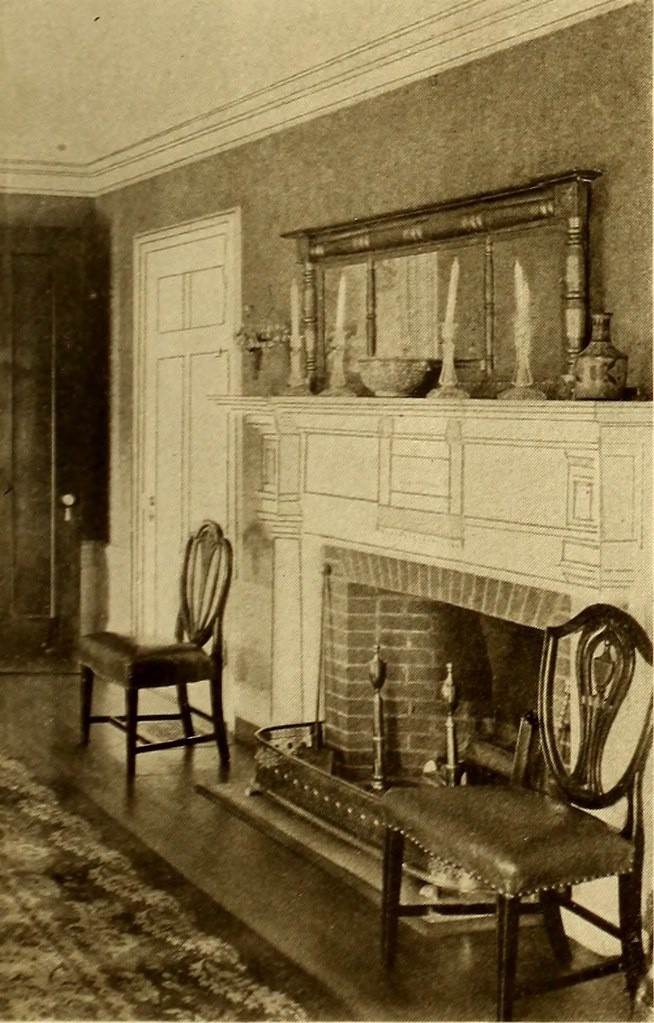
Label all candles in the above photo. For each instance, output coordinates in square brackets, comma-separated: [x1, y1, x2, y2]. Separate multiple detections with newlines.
[444, 254, 459, 337]
[335, 276, 346, 350]
[291, 278, 300, 346]
[514, 262, 535, 347]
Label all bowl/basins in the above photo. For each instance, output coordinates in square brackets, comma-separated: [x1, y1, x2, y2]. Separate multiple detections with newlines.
[358, 358, 433, 396]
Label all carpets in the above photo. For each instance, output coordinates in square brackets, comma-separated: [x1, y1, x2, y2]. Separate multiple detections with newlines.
[0, 722, 364, 1021]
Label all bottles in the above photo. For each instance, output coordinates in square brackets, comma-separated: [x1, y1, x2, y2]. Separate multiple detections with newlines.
[573, 314, 629, 399]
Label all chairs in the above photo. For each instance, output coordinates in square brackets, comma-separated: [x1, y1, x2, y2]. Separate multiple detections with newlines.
[382, 602, 654, 1022]
[79, 520, 234, 784]
